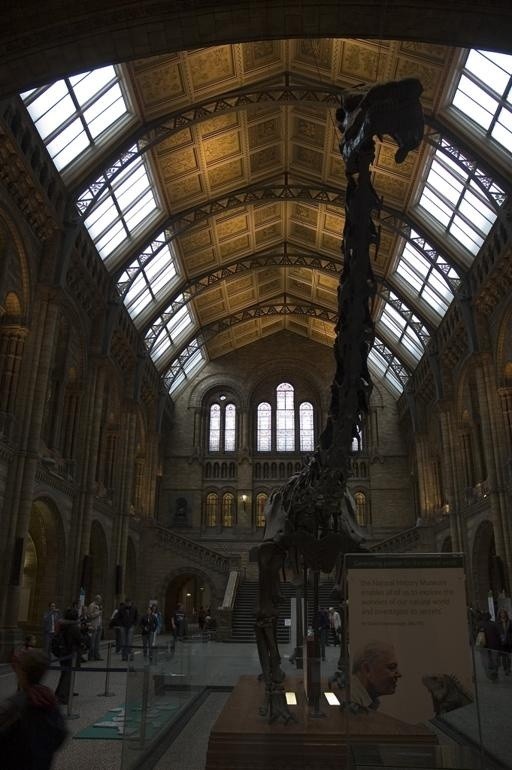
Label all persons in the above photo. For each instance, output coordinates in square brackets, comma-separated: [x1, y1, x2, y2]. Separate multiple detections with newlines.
[44, 593, 214, 667]
[348, 635, 404, 712]
[1, 648, 70, 770]
[52, 607, 87, 706]
[12, 633, 39, 692]
[466, 603, 511, 684]
[307, 606, 344, 648]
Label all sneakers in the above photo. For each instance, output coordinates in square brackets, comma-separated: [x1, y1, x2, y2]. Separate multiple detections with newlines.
[88, 656, 104, 661]
